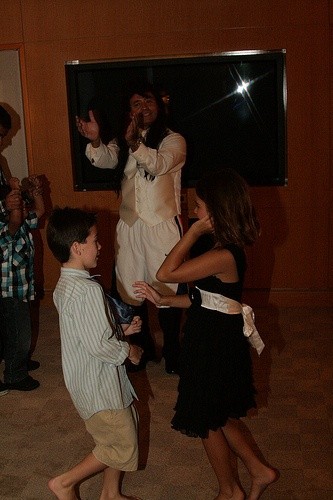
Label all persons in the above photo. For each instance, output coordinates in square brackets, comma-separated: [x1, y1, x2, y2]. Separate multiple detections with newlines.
[0, 102, 45, 397]
[44, 207, 142, 499]
[75, 85, 187, 375]
[131, 169, 278, 500]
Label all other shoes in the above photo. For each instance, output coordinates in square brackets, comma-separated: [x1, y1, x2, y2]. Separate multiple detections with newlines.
[128, 358, 146, 373]
[0, 382, 8, 396]
[165, 360, 177, 374]
[5, 376, 40, 391]
[28, 360, 40, 370]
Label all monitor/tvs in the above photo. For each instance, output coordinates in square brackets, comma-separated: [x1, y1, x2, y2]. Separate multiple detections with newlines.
[64, 48, 287, 192]
